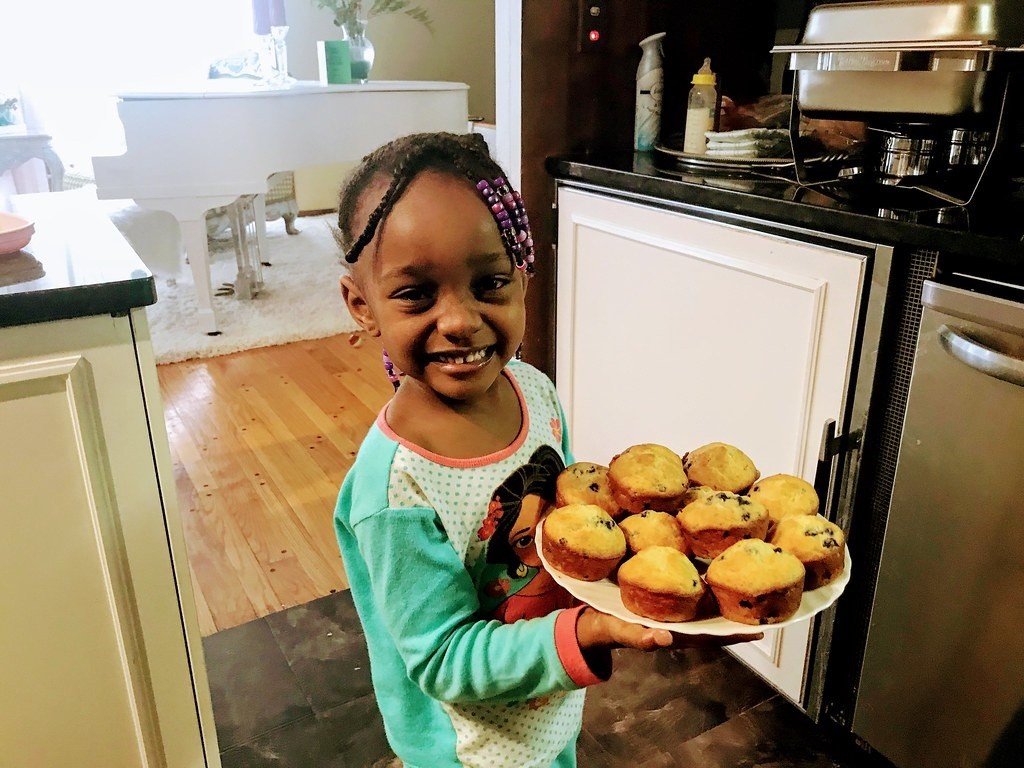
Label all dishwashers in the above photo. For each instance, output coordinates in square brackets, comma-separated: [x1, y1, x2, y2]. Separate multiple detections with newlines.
[849, 279, 1024, 768]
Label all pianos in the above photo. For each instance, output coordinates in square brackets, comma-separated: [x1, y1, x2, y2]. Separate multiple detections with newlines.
[91, 73, 472, 338]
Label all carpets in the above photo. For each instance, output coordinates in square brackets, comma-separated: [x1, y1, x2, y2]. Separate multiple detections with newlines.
[147, 211, 367, 365]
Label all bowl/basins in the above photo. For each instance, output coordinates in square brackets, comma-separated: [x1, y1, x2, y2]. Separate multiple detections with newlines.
[0, 209, 36, 256]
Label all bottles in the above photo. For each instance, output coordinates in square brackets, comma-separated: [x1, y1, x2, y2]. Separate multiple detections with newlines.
[632, 29, 674, 153]
[681, 57, 719, 155]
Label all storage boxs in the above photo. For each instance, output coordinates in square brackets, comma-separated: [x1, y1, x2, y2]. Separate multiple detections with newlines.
[317, 41, 352, 84]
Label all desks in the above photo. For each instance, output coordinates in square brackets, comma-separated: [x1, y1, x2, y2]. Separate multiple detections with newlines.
[0, 134, 65, 192]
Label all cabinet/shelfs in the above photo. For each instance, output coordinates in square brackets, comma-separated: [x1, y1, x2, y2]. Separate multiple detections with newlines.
[546, 176, 895, 727]
[0, 306, 222, 768]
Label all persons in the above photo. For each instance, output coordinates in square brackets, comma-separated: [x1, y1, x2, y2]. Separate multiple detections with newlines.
[336, 133, 764, 768]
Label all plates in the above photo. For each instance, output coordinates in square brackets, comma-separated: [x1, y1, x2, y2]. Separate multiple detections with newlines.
[677, 141, 837, 176]
[532, 497, 855, 637]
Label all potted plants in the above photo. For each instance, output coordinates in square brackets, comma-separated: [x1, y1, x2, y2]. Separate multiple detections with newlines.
[319, 0, 436, 84]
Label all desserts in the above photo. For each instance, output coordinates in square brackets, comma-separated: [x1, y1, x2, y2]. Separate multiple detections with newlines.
[543, 444, 845, 624]
